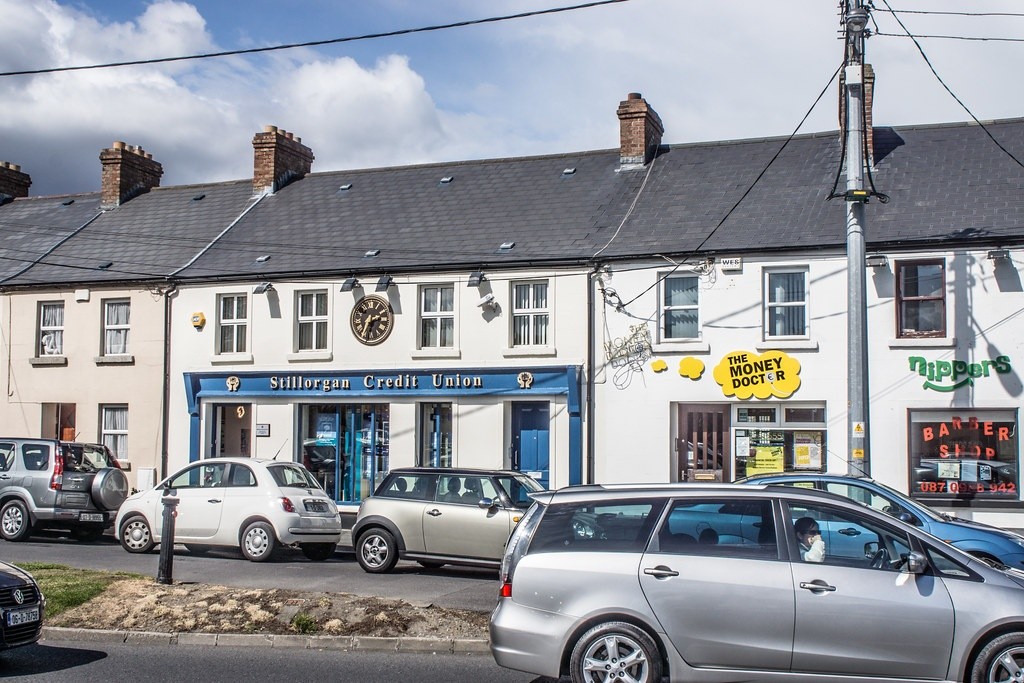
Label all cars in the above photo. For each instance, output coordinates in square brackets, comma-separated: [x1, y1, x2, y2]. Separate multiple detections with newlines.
[116, 458, 342, 559]
[0, 561, 46, 651]
[607, 471, 1024, 570]
[918, 458, 1018, 491]
[303, 439, 345, 493]
[349, 467, 606, 573]
[686, 442, 756, 477]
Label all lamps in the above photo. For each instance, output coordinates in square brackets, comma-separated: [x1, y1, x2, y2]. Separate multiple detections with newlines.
[467, 272, 483, 286]
[339, 279, 361, 291]
[375, 275, 395, 291]
[987, 250, 1010, 259]
[865, 255, 887, 266]
[252, 282, 275, 294]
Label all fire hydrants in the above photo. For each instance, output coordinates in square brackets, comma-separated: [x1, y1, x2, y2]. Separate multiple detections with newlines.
[157, 497, 180, 584]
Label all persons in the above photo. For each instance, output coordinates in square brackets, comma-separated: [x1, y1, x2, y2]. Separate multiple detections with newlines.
[794, 516, 825, 562]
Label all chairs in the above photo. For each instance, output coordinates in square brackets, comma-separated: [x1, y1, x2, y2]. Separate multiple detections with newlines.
[462, 476, 480, 505]
[444, 477, 463, 503]
[396, 478, 412, 498]
[669, 533, 698, 545]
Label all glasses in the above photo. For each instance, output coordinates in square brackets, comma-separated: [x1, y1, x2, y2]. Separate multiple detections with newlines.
[801, 529, 822, 535]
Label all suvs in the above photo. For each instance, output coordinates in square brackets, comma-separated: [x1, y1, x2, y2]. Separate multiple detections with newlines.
[0, 437, 129, 542]
[487, 483, 1024, 683]
